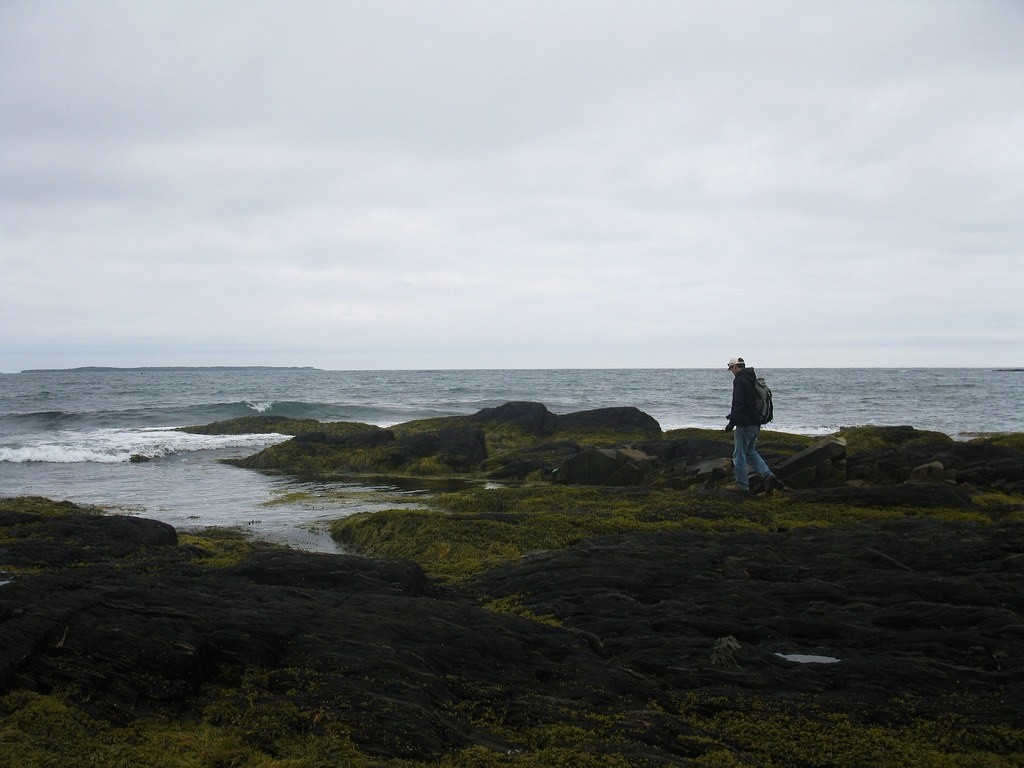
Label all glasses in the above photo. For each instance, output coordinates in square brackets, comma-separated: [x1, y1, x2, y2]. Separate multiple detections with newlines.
[728, 363, 734, 366]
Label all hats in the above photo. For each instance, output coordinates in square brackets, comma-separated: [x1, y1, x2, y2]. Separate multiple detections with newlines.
[727, 357, 745, 370]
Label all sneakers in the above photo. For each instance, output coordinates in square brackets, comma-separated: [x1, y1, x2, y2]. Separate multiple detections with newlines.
[763, 474, 777, 497]
[726, 485, 741, 493]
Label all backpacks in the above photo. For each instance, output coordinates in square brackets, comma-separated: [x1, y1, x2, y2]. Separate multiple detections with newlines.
[736, 373, 773, 424]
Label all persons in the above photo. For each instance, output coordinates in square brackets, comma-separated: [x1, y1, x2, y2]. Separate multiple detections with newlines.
[725, 357, 776, 494]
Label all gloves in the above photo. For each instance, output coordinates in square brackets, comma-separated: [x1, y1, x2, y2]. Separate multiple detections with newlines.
[725, 414, 734, 432]
